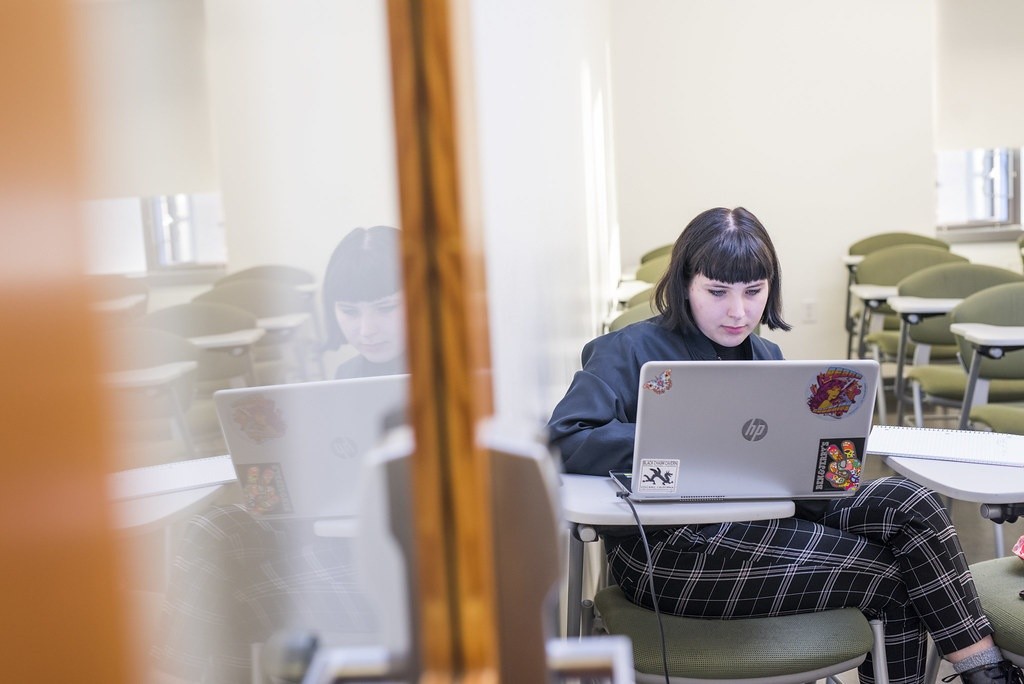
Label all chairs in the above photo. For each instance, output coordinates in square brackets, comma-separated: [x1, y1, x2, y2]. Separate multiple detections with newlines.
[845, 233, 1024, 669]
[97, 263, 322, 535]
[579, 243, 888, 684]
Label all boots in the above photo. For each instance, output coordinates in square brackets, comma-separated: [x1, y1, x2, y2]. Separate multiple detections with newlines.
[960, 660, 1021, 684]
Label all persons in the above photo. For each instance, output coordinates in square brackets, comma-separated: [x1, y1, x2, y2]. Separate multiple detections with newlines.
[322, 225, 410, 381]
[547, 206, 1024, 683]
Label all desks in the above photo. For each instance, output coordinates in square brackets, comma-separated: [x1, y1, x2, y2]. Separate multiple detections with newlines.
[111, 485, 231, 532]
[849, 285, 900, 300]
[880, 455, 1023, 505]
[101, 361, 199, 384]
[99, 295, 148, 314]
[256, 313, 312, 330]
[145, 286, 218, 308]
[561, 472, 797, 526]
[187, 327, 267, 347]
[948, 323, 1024, 348]
[887, 296, 969, 316]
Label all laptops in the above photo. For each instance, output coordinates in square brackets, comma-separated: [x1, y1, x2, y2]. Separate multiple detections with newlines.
[213, 373, 417, 523]
[609, 359, 879, 501]
